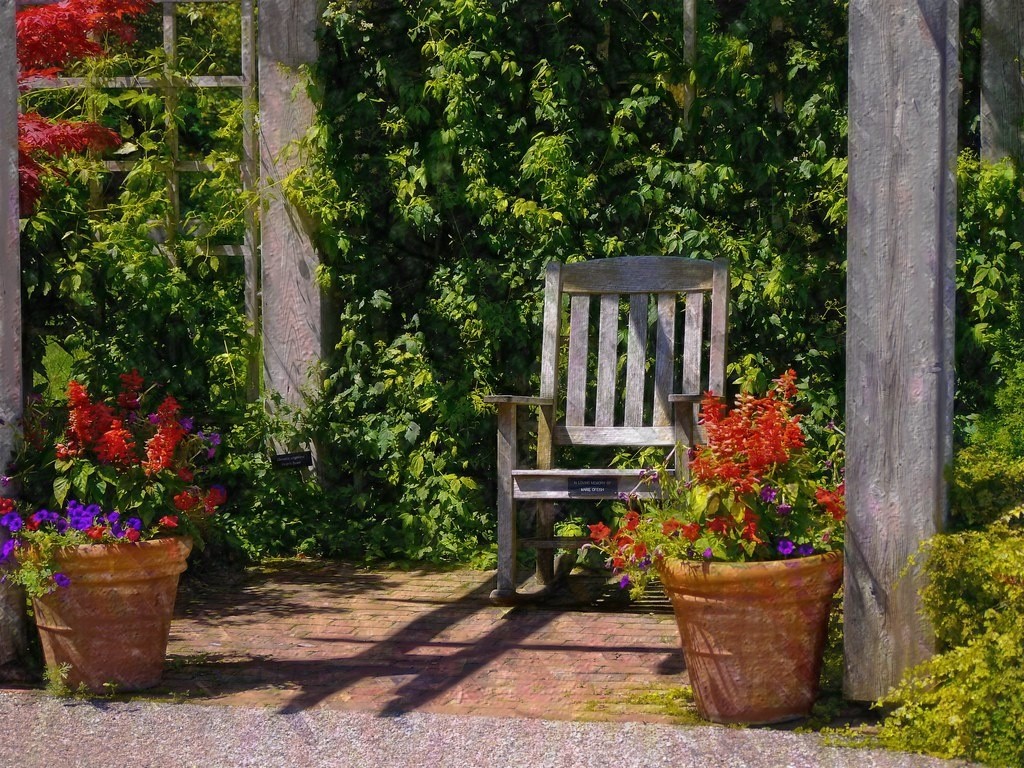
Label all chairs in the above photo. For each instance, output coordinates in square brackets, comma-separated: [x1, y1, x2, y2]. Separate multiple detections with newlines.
[485, 255, 730, 606]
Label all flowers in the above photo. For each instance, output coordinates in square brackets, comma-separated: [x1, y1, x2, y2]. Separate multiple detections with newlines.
[0, 372, 230, 616]
[576, 354, 847, 598]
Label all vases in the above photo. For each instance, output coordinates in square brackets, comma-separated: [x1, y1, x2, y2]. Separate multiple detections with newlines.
[657, 555, 847, 726]
[16, 536, 192, 691]
